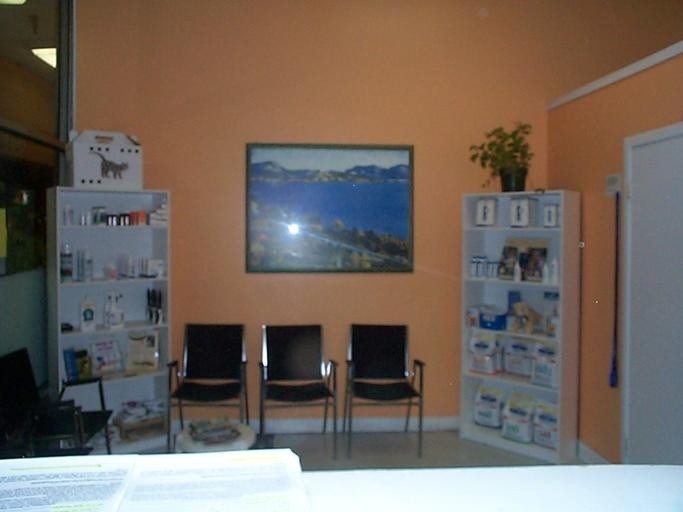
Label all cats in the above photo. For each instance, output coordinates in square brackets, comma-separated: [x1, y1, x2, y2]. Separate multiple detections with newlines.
[89, 149, 130, 179]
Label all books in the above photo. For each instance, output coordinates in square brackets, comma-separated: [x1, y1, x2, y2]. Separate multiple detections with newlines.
[91, 341, 124, 377]
[126, 332, 159, 370]
[63, 345, 78, 384]
[74, 349, 89, 382]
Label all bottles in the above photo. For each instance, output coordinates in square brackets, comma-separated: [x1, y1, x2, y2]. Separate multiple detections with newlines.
[59, 238, 74, 285]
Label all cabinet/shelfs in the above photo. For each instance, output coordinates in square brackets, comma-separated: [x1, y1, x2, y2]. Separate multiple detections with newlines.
[46, 185, 171, 454]
[460, 190, 582, 464]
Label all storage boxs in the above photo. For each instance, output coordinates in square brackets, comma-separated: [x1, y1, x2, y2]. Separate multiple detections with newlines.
[113, 415, 169, 442]
[66, 130, 143, 187]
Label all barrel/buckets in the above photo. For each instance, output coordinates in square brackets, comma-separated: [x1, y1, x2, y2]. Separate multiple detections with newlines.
[38, 433, 79, 456]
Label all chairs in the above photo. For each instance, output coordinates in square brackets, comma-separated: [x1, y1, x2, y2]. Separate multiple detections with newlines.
[341, 324, 425, 458]
[0, 346, 113, 458]
[259, 324, 337, 456]
[168, 323, 249, 445]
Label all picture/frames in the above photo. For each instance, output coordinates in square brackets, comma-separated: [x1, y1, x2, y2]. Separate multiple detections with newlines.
[246, 143, 414, 272]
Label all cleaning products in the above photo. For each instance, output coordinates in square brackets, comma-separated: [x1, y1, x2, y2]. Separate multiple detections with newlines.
[103, 290, 127, 330]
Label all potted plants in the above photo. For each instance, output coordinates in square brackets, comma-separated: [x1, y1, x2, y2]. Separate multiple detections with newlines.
[469, 122, 534, 192]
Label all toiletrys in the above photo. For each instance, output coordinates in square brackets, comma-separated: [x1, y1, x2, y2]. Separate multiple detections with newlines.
[63, 204, 147, 226]
[59, 240, 73, 277]
[82, 297, 97, 331]
[58, 242, 135, 282]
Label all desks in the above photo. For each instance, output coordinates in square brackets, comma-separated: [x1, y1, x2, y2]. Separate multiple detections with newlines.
[0, 464, 683, 512]
